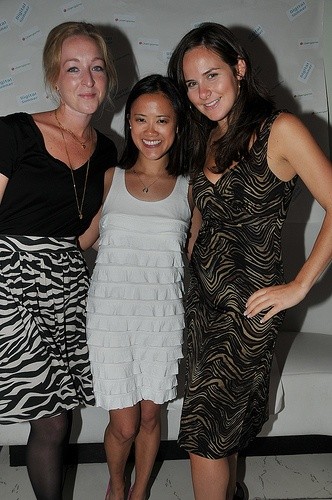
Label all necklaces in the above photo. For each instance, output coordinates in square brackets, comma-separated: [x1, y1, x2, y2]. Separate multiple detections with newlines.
[54, 110, 93, 219]
[59, 125, 90, 149]
[133, 165, 168, 193]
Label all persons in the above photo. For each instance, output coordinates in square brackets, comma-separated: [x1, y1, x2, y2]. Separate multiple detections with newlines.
[77, 73, 203, 500]
[0, 21, 118, 500]
[173, 22, 332, 500]
